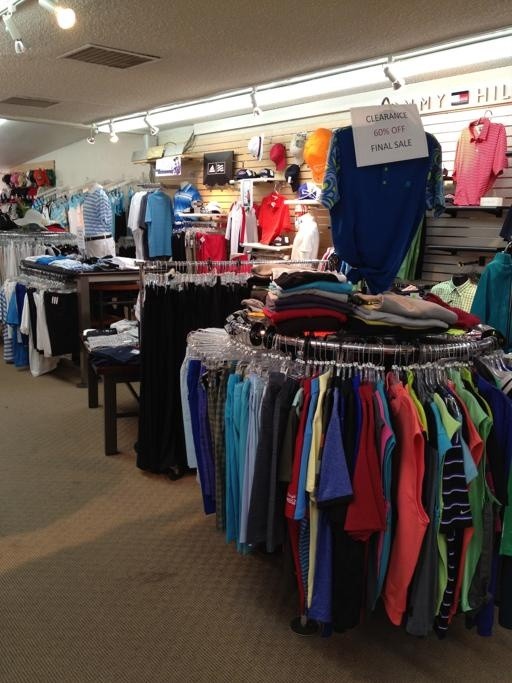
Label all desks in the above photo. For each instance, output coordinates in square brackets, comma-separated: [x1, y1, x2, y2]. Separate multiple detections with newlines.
[74, 269, 141, 456]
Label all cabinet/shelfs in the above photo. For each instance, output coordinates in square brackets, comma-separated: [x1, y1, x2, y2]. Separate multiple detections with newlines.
[427, 202, 510, 255]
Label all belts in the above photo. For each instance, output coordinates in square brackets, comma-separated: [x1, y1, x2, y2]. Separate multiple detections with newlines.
[84, 234, 112, 242]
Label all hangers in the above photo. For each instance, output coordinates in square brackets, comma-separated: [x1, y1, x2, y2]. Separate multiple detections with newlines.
[143, 258, 262, 291]
[14, 266, 67, 293]
[451, 263, 484, 282]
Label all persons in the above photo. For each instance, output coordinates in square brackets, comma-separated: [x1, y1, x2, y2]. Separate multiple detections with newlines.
[172, 180, 202, 230]
[82, 182, 116, 259]
[291, 204, 319, 263]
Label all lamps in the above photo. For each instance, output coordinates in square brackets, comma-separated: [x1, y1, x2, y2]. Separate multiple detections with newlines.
[88, 52, 407, 145]
[0, 0, 77, 55]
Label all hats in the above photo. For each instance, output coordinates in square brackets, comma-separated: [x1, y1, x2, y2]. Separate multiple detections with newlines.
[290, 129, 306, 165]
[206, 201, 221, 213]
[304, 127, 333, 183]
[285, 164, 300, 192]
[270, 143, 287, 171]
[246, 134, 263, 160]
